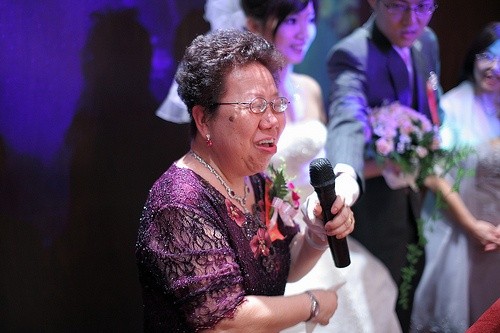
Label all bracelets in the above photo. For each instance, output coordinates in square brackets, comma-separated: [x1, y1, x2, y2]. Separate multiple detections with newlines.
[304, 228, 328, 251]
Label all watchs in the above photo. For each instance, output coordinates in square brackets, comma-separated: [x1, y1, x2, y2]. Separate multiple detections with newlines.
[304, 291, 319, 322]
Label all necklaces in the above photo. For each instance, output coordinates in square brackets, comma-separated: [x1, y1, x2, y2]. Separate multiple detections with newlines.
[190, 151, 249, 214]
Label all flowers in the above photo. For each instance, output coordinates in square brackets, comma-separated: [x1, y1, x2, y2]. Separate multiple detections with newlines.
[269, 163, 300, 218]
[364, 101, 478, 311]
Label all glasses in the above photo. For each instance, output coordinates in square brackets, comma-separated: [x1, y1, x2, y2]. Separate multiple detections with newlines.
[382, 0, 438, 15]
[474, 51, 500, 67]
[211, 97, 290, 114]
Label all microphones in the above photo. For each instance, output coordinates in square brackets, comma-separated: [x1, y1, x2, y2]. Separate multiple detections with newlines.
[309, 158, 351, 268]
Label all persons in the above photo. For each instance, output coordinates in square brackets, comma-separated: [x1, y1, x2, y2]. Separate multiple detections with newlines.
[423, 22, 500, 333]
[135, 29, 355, 333]
[211, 0, 402, 333]
[325, 0, 444, 333]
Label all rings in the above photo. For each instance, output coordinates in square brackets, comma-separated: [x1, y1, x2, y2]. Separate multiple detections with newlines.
[349, 217, 353, 224]
